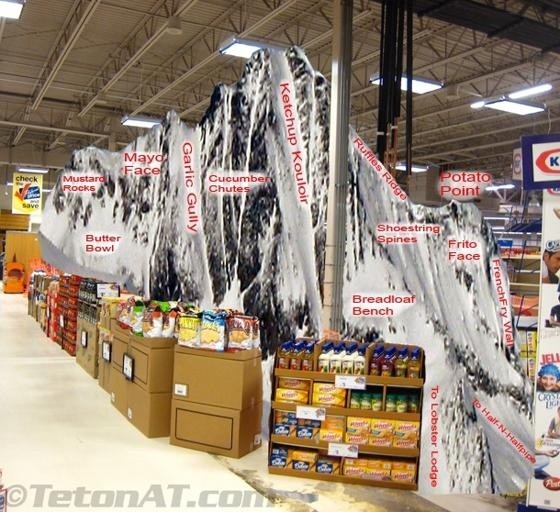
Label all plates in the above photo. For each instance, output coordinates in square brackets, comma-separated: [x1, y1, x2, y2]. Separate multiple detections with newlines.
[268, 337, 426, 490]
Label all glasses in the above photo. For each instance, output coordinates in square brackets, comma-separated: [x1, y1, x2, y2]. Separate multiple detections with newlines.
[16, 166, 49, 174]
[390, 161, 429, 173]
[121, 114, 162, 129]
[484, 96, 544, 116]
[219, 36, 283, 59]
[0, 0, 26, 20]
[369, 71, 445, 95]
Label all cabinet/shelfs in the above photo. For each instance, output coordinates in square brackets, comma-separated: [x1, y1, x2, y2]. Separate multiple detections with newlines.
[350, 393, 418, 413]
[278, 340, 421, 378]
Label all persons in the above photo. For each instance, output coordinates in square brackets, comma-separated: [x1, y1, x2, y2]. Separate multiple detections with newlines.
[547, 406, 560, 439]
[534, 447, 560, 458]
[536, 363, 560, 392]
[542, 239, 560, 284]
[549, 294, 560, 327]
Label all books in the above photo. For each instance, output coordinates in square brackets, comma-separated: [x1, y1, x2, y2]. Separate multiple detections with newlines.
[76, 317, 264, 459]
